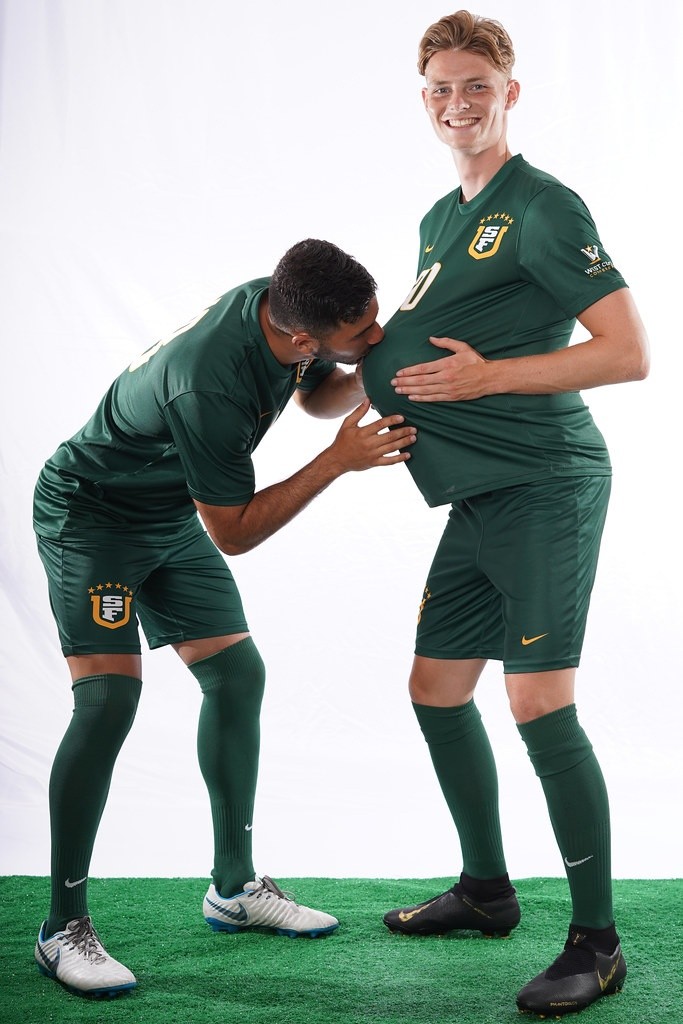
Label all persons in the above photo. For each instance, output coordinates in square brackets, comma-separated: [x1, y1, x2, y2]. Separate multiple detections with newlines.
[32, 238, 418, 994]
[362, 10, 648, 1019]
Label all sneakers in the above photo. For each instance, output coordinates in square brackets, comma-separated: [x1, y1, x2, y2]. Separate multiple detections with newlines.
[515, 933, 627, 1017]
[203, 874, 339, 939]
[32, 916, 136, 997]
[382, 882, 521, 938]
